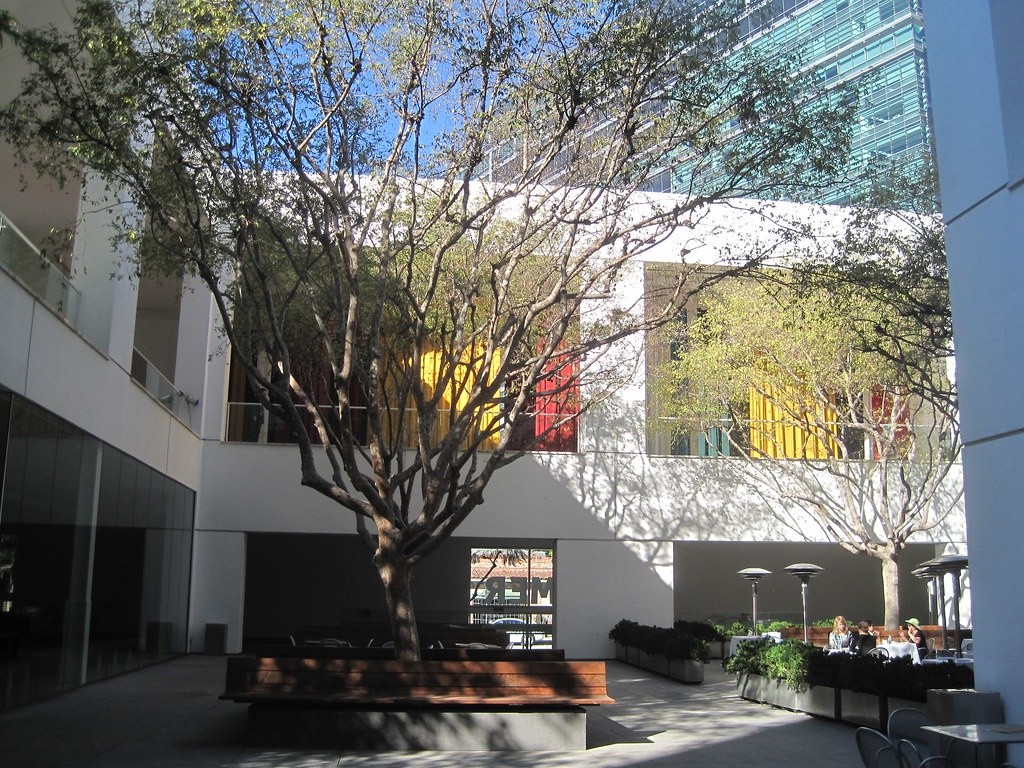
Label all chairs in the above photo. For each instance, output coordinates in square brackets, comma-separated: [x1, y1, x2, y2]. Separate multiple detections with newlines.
[348, 638, 394, 647]
[762, 631, 781, 643]
[427, 640, 487, 649]
[827, 632, 973, 660]
[855, 708, 948, 768]
[290, 635, 295, 646]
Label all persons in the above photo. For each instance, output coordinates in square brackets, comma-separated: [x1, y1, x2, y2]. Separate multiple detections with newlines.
[900, 617, 927, 659]
[849, 622, 876, 655]
[829, 616, 852, 649]
[863, 619, 881, 645]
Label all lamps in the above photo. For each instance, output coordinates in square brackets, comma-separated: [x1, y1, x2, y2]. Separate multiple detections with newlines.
[185, 394, 200, 406]
[735, 567, 772, 636]
[911, 553, 972, 658]
[784, 563, 824, 644]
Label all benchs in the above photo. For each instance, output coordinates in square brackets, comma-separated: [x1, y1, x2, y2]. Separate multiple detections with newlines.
[235, 645, 616, 752]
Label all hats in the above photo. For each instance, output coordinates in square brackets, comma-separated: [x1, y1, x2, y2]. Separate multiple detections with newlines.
[905, 618, 921, 631]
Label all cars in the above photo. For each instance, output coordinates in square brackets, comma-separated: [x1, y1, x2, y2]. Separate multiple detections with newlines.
[491, 618, 545, 646]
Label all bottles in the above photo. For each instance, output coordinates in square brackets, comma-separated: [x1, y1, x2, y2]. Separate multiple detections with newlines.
[887, 636, 892, 644]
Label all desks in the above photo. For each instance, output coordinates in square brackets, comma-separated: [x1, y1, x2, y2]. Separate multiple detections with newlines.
[304, 640, 346, 646]
[878, 641, 921, 664]
[456, 643, 502, 649]
[921, 723, 1024, 768]
[829, 648, 857, 655]
[730, 635, 781, 657]
[923, 657, 974, 669]
